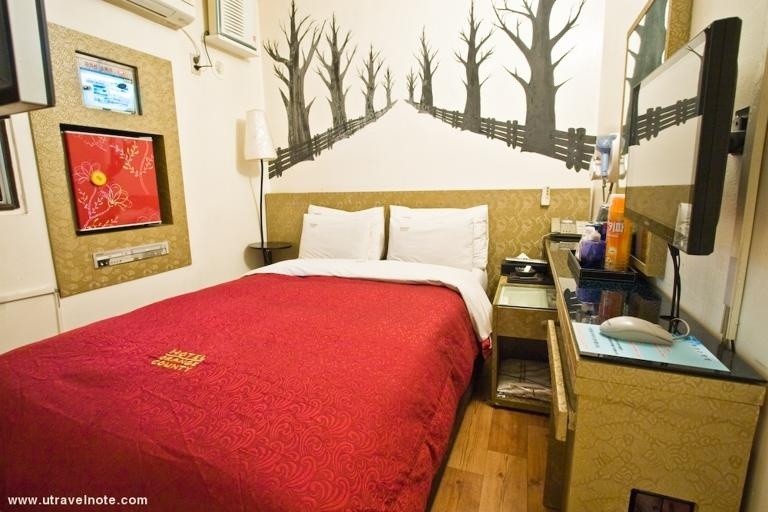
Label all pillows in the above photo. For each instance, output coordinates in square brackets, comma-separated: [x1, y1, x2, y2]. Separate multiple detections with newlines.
[298, 202, 489, 272]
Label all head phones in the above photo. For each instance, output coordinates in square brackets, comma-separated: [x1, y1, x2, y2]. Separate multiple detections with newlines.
[596, 134, 617, 204]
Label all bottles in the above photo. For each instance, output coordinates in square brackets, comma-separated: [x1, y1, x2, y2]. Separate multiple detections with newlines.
[575, 286, 624, 326]
[575, 194, 633, 274]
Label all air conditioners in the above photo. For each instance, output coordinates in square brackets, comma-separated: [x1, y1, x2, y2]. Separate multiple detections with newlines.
[105, 0, 198, 30]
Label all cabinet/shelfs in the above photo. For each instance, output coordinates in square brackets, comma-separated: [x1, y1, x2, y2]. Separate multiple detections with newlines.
[490, 238, 767, 511]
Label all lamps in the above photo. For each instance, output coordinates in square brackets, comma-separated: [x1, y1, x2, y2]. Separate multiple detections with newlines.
[242, 109, 291, 250]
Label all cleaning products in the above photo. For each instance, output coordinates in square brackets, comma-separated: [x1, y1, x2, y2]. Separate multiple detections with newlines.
[605, 193, 625, 270]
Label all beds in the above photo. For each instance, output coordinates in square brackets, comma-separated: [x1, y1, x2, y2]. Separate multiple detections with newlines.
[1, 257, 488, 512]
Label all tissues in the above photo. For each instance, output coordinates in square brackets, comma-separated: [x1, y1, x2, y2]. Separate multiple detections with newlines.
[500, 252, 549, 276]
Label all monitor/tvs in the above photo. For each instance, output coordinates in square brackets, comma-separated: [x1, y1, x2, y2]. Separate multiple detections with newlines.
[623, 16, 743, 255]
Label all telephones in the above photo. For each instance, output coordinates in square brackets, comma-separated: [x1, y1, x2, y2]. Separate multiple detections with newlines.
[551, 218, 577, 234]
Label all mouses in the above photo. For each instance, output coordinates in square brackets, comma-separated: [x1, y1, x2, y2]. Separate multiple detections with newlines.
[599, 315, 674, 346]
